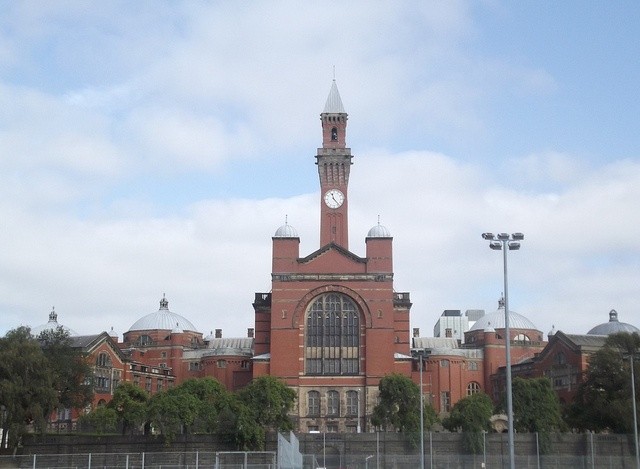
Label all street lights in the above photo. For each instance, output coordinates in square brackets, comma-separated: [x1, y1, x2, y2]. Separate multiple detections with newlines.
[481, 231, 524, 468]
[410, 346, 434, 469]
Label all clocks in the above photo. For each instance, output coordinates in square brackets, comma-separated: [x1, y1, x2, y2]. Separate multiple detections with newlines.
[324, 188, 345, 209]
